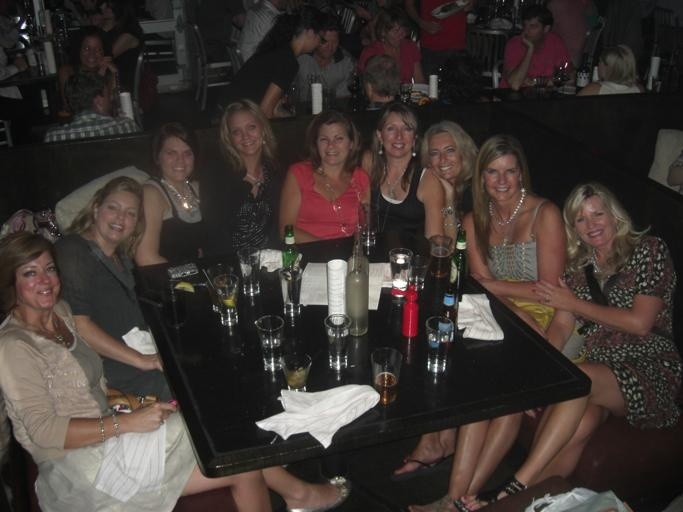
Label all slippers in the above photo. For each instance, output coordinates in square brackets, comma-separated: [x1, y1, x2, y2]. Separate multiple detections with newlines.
[392, 455, 441, 477]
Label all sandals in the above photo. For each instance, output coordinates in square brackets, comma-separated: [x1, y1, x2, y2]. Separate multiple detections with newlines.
[451, 474, 528, 511]
[407, 494, 455, 512]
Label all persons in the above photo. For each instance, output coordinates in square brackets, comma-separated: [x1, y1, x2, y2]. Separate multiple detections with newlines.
[0, 0, 683, 512]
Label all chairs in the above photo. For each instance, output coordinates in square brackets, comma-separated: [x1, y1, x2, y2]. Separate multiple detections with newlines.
[463, 28, 508, 89]
[181, 21, 244, 111]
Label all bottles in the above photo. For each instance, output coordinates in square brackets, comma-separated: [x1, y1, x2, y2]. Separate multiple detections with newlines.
[574, 51, 591, 93]
[280, 222, 300, 273]
[346, 222, 370, 336]
[402, 225, 470, 349]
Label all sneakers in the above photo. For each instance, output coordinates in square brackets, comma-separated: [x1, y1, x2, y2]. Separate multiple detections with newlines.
[286, 475, 353, 512]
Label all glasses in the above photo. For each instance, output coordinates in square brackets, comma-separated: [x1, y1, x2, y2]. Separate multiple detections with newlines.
[80, 45, 102, 55]
[315, 32, 329, 45]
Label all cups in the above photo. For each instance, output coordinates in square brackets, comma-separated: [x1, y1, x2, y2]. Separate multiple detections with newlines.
[426, 315, 454, 375]
[388, 229, 450, 291]
[324, 314, 353, 354]
[281, 350, 312, 393]
[206, 247, 301, 351]
[357, 181, 382, 245]
[370, 347, 404, 407]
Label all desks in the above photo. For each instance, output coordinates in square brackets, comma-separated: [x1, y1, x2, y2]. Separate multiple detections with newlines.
[129, 229, 592, 479]
